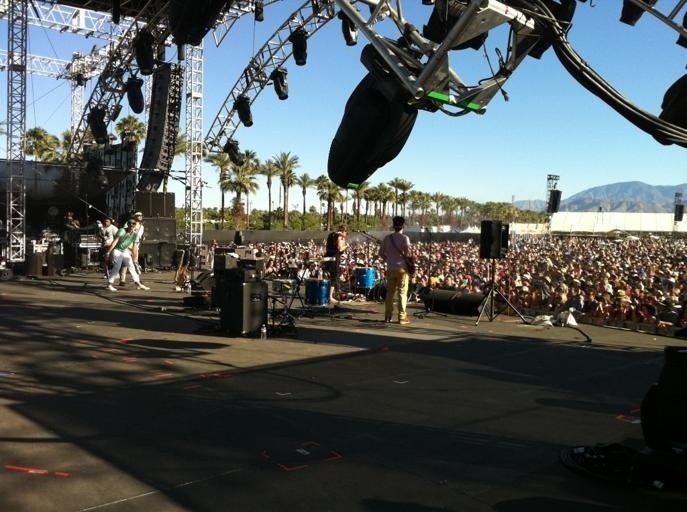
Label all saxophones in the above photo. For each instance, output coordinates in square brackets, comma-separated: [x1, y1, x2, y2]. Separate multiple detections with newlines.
[174, 249, 185, 281]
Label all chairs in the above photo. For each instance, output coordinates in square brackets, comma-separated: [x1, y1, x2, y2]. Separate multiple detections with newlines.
[268, 265, 308, 335]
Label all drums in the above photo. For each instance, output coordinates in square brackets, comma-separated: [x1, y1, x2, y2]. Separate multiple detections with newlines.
[272, 279, 297, 295]
[304, 278, 331, 306]
[353, 267, 375, 289]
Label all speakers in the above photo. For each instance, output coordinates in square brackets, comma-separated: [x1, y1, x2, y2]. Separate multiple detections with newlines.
[659, 345, 687, 450]
[479, 221, 509, 259]
[549, 190, 562, 213]
[676, 204, 684, 221]
[214, 247, 268, 337]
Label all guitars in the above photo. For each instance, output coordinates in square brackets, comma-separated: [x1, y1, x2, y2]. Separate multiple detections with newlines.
[376, 239, 418, 278]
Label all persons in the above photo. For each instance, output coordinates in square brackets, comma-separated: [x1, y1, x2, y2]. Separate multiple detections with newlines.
[327, 224, 350, 304]
[379, 216, 410, 324]
[58, 212, 80, 273]
[96, 212, 150, 291]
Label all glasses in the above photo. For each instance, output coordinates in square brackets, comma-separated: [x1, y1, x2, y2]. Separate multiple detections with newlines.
[127, 222, 136, 225]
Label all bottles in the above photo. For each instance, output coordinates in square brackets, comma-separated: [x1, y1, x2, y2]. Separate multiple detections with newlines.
[260, 324, 267, 341]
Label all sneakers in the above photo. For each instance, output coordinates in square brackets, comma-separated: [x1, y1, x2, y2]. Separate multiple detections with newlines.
[399, 318, 410, 324]
[136, 284, 150, 290]
[105, 284, 117, 292]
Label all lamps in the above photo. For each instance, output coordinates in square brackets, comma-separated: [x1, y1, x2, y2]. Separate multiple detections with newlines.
[222, 8, 359, 165]
[86, 31, 154, 144]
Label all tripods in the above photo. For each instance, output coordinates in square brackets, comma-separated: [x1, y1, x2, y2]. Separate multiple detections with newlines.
[476, 259, 527, 326]
